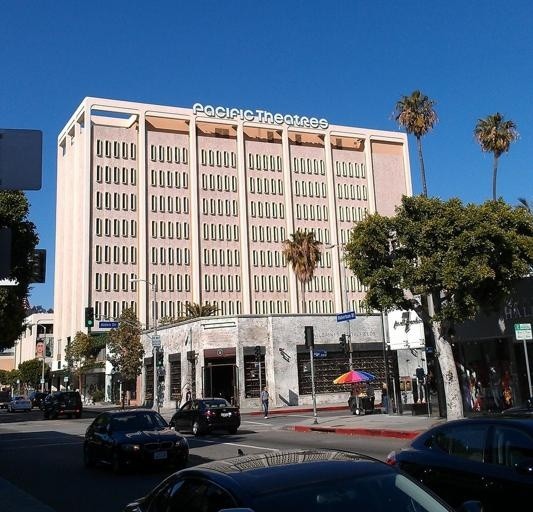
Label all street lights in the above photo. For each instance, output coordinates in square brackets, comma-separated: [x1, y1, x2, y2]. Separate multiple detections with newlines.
[27, 322, 47, 392]
[126, 278, 158, 413]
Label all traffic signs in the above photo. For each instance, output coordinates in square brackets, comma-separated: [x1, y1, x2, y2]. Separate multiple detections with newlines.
[98, 321, 118, 328]
[336, 311, 355, 322]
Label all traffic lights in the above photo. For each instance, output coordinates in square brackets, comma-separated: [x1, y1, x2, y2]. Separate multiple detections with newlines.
[337, 333, 345, 351]
[84, 306, 94, 327]
[156, 352, 163, 366]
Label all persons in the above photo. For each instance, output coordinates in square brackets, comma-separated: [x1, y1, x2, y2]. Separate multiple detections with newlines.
[259, 386, 269, 419]
[365, 381, 375, 414]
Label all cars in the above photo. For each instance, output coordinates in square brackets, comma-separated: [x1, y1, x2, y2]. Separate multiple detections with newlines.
[122, 447, 483, 510]
[168, 397, 240, 437]
[82, 406, 188, 477]
[382, 411, 532, 511]
[0, 390, 83, 420]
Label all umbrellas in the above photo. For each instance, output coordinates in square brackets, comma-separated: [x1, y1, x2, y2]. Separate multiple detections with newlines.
[331, 369, 376, 408]
